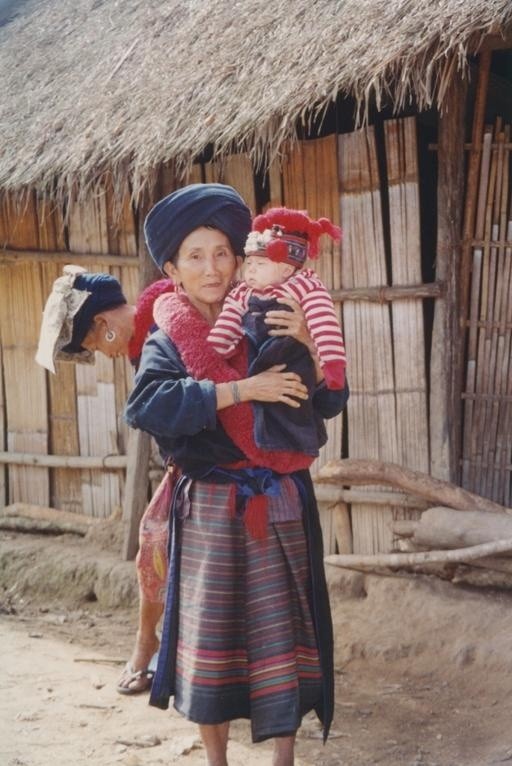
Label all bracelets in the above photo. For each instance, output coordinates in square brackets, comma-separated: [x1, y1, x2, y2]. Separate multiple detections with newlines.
[233, 379, 241, 405]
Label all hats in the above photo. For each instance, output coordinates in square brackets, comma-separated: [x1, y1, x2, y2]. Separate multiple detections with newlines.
[56, 272, 128, 356]
[141, 180, 255, 277]
[243, 206, 344, 270]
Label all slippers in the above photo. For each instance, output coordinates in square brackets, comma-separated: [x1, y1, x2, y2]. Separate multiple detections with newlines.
[113, 660, 158, 697]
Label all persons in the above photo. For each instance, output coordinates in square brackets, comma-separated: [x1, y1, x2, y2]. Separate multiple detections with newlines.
[127, 184, 347, 766]
[35, 266, 201, 696]
[202, 208, 348, 439]
